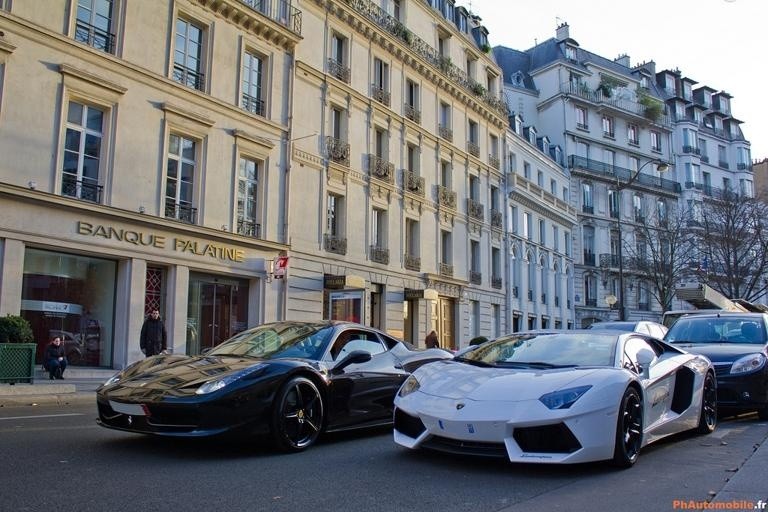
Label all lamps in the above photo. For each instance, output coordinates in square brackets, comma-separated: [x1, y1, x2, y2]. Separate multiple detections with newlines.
[30, 182, 37, 189]
[222, 224, 228, 230]
[138, 207, 146, 213]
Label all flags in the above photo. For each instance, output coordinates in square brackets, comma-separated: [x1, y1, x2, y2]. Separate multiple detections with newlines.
[272, 257, 288, 281]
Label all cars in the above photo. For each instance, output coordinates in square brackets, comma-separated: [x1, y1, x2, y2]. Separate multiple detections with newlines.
[48, 330, 99, 365]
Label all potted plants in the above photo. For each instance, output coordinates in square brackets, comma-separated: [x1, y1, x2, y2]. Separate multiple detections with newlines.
[0, 313, 37, 386]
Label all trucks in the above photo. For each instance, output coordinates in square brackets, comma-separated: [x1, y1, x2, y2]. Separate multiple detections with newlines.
[661, 282, 768, 329]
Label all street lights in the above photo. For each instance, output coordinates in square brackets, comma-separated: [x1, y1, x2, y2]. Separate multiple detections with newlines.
[616, 158, 669, 321]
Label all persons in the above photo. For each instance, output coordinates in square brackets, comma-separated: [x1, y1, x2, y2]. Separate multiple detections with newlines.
[424, 330, 440, 349]
[139, 308, 167, 357]
[41, 335, 67, 380]
